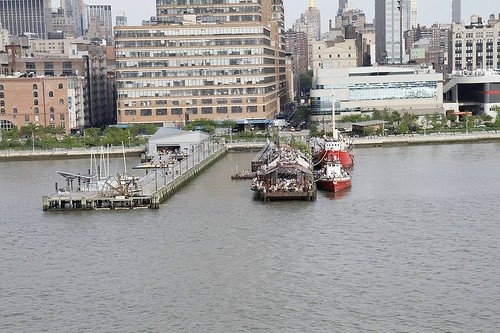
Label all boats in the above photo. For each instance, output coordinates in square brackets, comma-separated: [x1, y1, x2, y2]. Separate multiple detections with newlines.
[229, 94, 355, 203]
